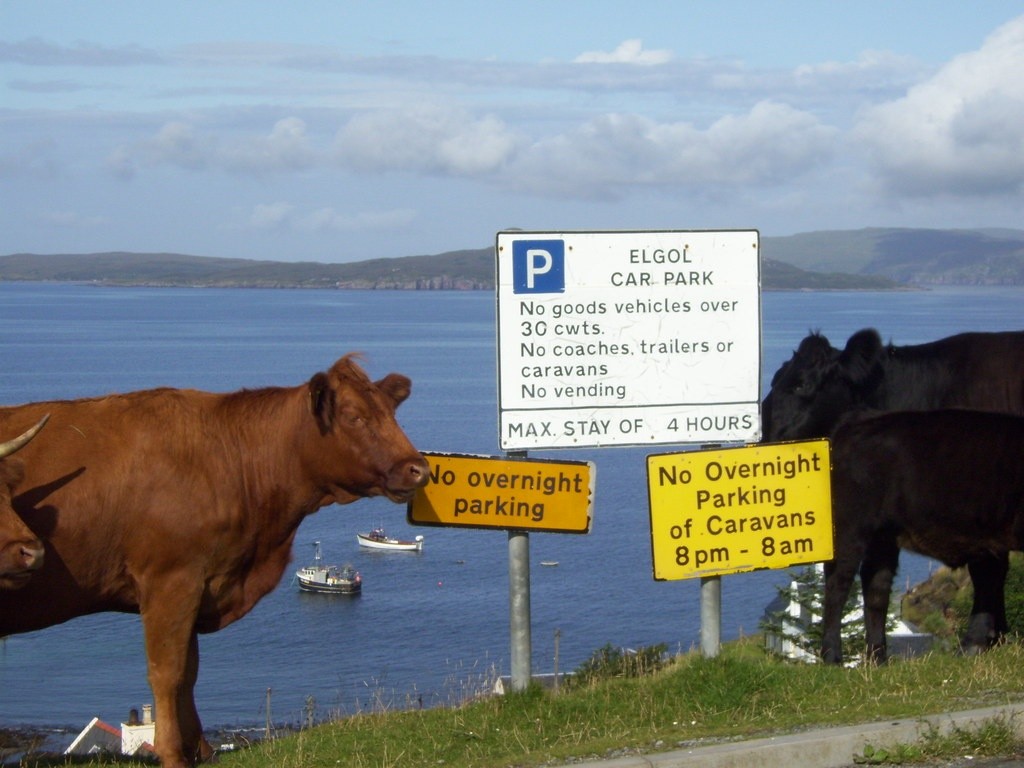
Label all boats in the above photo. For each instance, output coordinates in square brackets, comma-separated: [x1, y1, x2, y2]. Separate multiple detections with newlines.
[294, 538, 363, 596]
[354, 517, 426, 552]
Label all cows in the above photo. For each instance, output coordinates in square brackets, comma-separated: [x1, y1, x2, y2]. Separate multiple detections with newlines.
[0, 412, 54, 594]
[0, 352, 431, 768]
[759, 325, 1024, 666]
